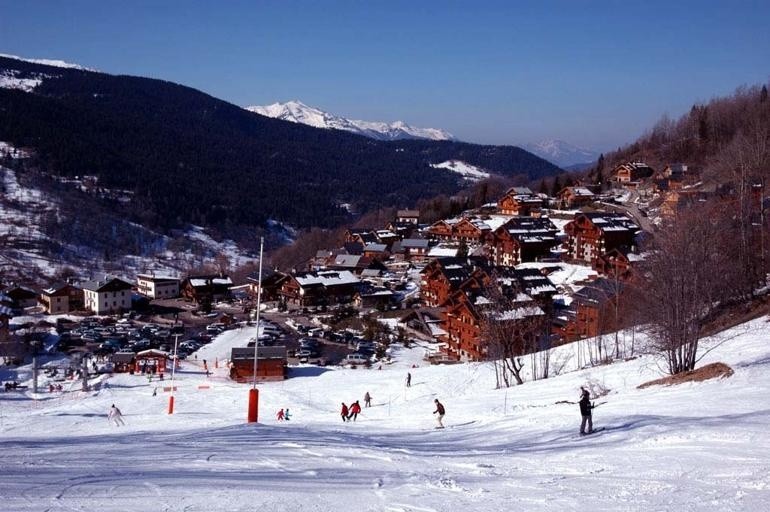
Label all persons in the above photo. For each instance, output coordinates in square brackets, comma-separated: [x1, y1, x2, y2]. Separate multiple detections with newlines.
[277, 409, 284, 420]
[129, 361, 163, 381]
[433, 399, 446, 427]
[579, 390, 595, 435]
[364, 391, 373, 408]
[406, 373, 411, 387]
[108, 404, 125, 426]
[580, 386, 584, 398]
[347, 400, 361, 421]
[5, 361, 98, 392]
[340, 402, 350, 421]
[152, 387, 157, 396]
[284, 408, 292, 420]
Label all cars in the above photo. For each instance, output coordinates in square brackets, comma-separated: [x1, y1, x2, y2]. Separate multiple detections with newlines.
[249, 319, 388, 366]
[63, 314, 227, 363]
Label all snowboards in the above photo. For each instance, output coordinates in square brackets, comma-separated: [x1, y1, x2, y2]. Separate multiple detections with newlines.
[423, 425, 453, 430]
[572, 426, 605, 439]
